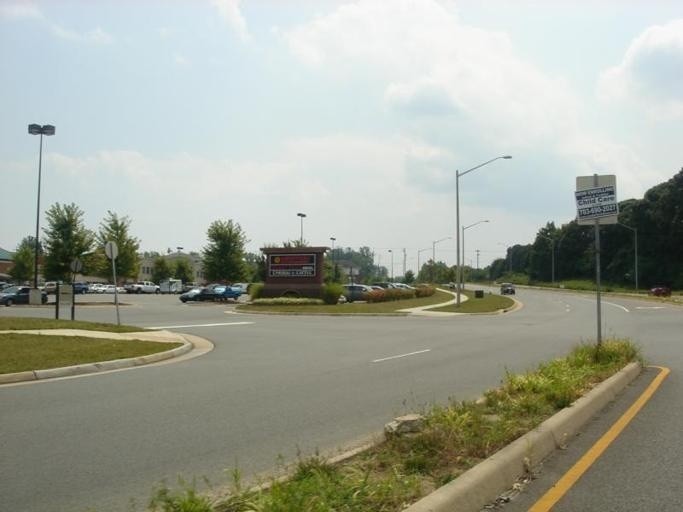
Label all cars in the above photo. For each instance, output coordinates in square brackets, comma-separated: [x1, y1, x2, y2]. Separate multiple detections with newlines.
[647, 286, 671, 297]
[0, 274, 126, 307]
[179, 279, 252, 303]
[339, 283, 415, 303]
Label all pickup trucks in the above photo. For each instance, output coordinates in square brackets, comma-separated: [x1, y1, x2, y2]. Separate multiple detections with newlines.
[127, 281, 160, 295]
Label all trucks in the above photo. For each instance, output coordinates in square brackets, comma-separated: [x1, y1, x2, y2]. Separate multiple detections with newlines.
[159, 278, 181, 293]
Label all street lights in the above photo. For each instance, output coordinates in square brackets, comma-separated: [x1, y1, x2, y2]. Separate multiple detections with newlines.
[28, 122, 55, 288]
[461, 220, 488, 291]
[330, 237, 335, 264]
[454, 155, 511, 311]
[387, 250, 393, 282]
[297, 213, 306, 246]
[432, 236, 451, 264]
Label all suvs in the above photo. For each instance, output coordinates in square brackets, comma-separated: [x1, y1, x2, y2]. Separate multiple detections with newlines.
[500, 283, 515, 295]
